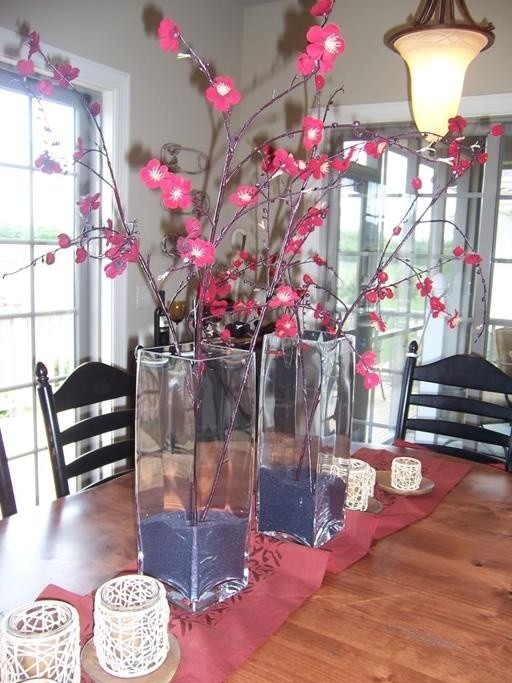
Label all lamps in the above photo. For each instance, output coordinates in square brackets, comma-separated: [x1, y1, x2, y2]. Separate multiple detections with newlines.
[382, 0, 496, 143]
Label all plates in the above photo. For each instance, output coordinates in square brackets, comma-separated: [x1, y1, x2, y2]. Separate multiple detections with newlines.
[79, 634, 182, 683]
[375, 471, 435, 495]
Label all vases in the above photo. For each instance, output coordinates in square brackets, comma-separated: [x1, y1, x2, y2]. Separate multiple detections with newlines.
[255, 326, 358, 550]
[130, 342, 257, 611]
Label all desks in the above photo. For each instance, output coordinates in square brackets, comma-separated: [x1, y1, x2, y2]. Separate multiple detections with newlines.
[1, 429, 512, 683]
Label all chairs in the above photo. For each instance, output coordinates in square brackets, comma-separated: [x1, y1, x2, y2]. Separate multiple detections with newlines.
[394, 339, 512, 468]
[0, 431, 23, 520]
[34, 360, 136, 499]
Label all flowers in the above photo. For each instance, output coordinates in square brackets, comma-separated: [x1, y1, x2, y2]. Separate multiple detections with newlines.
[1, 1, 512, 531]
